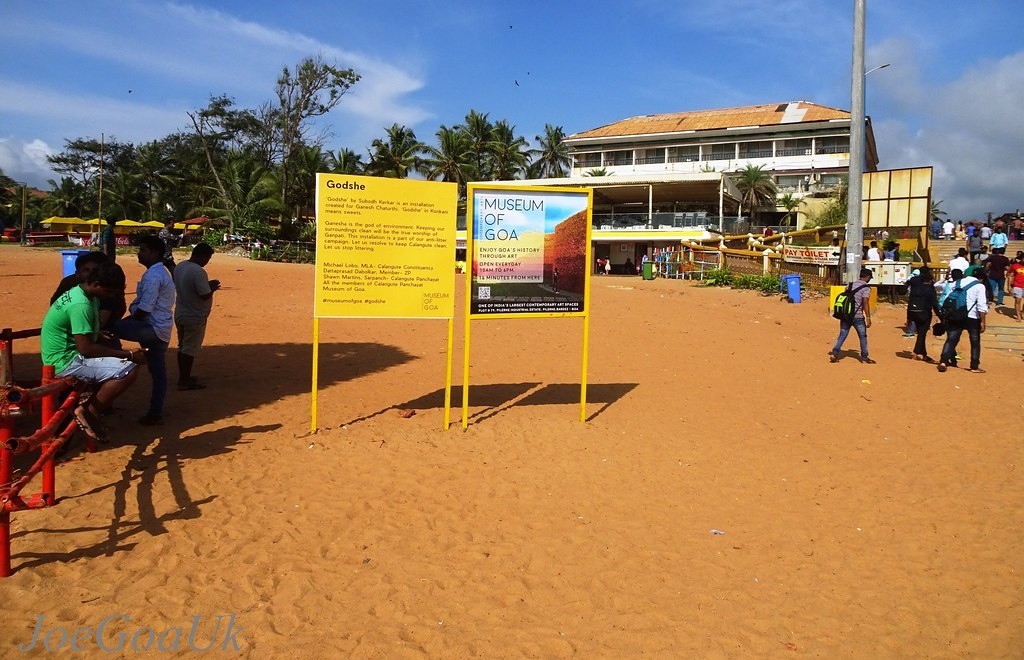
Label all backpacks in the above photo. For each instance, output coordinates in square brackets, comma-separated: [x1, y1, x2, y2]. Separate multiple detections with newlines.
[907, 287, 930, 322]
[941, 279, 980, 321]
[833, 282, 869, 324]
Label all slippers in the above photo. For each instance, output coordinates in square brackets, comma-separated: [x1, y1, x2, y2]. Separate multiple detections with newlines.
[74, 406, 113, 443]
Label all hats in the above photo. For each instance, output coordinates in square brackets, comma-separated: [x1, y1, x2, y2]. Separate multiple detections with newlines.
[913, 269, 920, 275]
[861, 269, 873, 278]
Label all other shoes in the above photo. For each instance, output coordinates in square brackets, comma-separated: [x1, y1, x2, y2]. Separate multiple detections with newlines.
[971, 369, 985, 373]
[862, 359, 877, 364]
[139, 415, 163, 425]
[830, 354, 839, 363]
[177, 376, 206, 391]
[912, 354, 932, 362]
[902, 334, 914, 338]
[939, 363, 947, 372]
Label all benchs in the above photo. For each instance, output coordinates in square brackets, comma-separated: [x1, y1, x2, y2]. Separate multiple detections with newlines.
[612, 264, 634, 274]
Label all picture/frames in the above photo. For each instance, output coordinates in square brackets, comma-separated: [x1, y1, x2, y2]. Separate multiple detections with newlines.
[620, 243, 628, 252]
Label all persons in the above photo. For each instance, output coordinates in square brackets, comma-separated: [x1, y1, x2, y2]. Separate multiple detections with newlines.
[902, 229, 910, 239]
[50, 251, 126, 329]
[764, 226, 773, 245]
[159, 219, 176, 277]
[20, 228, 26, 246]
[604, 256, 611, 275]
[966, 229, 983, 263]
[552, 268, 559, 294]
[223, 230, 229, 253]
[104, 235, 176, 426]
[866, 240, 899, 299]
[99, 215, 116, 262]
[904, 268, 988, 373]
[946, 246, 1024, 322]
[989, 226, 1008, 255]
[173, 243, 221, 390]
[931, 215, 1024, 240]
[624, 258, 634, 274]
[829, 268, 875, 363]
[40, 263, 149, 444]
[828, 237, 841, 277]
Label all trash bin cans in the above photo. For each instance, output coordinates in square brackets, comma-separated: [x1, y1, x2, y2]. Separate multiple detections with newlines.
[781, 274, 802, 303]
[643, 261, 653, 280]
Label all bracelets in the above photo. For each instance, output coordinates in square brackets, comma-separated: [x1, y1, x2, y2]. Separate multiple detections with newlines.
[129, 350, 134, 360]
